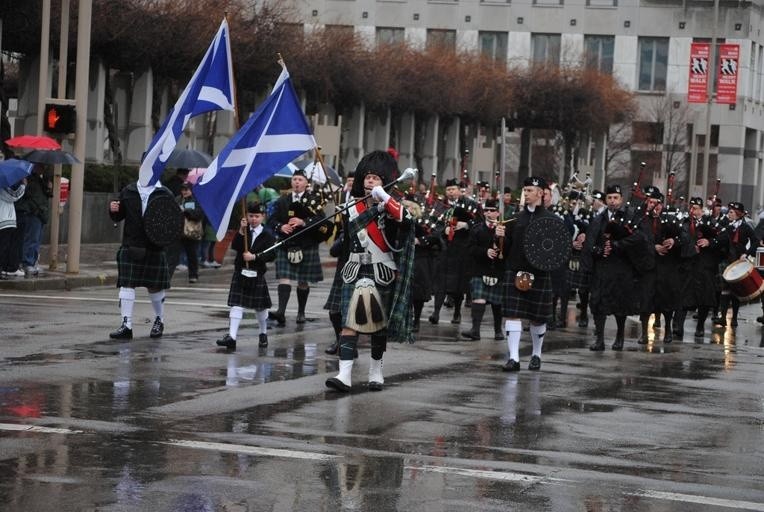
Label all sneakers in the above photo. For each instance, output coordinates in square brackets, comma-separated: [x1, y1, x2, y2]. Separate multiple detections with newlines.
[502, 359, 520, 372]
[200, 260, 222, 268]
[259, 333, 267, 347]
[216, 335, 236, 348]
[110, 325, 133, 339]
[150, 321, 164, 338]
[529, 355, 540, 370]
[1, 265, 39, 277]
[189, 278, 197, 283]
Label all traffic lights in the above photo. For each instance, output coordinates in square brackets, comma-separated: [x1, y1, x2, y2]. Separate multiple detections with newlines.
[40, 101, 77, 135]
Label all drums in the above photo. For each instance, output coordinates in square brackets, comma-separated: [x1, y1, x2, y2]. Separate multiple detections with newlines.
[755, 247, 764, 271]
[722, 259, 764, 301]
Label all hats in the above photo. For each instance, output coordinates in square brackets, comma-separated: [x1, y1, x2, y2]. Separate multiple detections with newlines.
[181, 181, 192, 189]
[480, 181, 490, 188]
[248, 201, 265, 214]
[446, 177, 460, 187]
[293, 169, 307, 179]
[504, 186, 511, 194]
[485, 198, 499, 208]
[350, 150, 403, 198]
[569, 184, 745, 211]
[177, 168, 191, 172]
[523, 177, 546, 189]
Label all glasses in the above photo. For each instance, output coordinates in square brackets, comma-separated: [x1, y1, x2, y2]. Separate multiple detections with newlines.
[484, 209, 497, 212]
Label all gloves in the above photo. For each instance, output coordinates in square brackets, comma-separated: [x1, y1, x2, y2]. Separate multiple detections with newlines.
[371, 185, 391, 205]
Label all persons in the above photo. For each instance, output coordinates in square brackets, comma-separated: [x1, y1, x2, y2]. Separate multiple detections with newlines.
[0, 162, 54, 277]
[213, 147, 764, 391]
[109, 172, 222, 342]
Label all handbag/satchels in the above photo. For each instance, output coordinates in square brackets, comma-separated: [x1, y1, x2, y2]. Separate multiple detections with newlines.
[515, 271, 535, 291]
[183, 217, 203, 241]
[287, 247, 303, 264]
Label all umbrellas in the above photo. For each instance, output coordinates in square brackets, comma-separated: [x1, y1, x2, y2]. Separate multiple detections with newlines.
[3, 135, 62, 151]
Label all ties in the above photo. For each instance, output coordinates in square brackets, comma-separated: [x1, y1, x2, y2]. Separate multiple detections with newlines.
[250, 229, 255, 247]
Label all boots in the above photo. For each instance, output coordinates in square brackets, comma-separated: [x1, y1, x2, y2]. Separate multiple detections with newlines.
[413, 294, 504, 340]
[268, 284, 291, 323]
[590, 318, 605, 351]
[325, 359, 354, 391]
[578, 291, 588, 328]
[638, 306, 739, 347]
[612, 315, 626, 350]
[552, 295, 569, 328]
[368, 355, 384, 390]
[325, 311, 342, 355]
[296, 286, 309, 323]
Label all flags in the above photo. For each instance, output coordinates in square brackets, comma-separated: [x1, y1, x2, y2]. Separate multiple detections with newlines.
[138, 18, 235, 187]
[190, 64, 316, 242]
